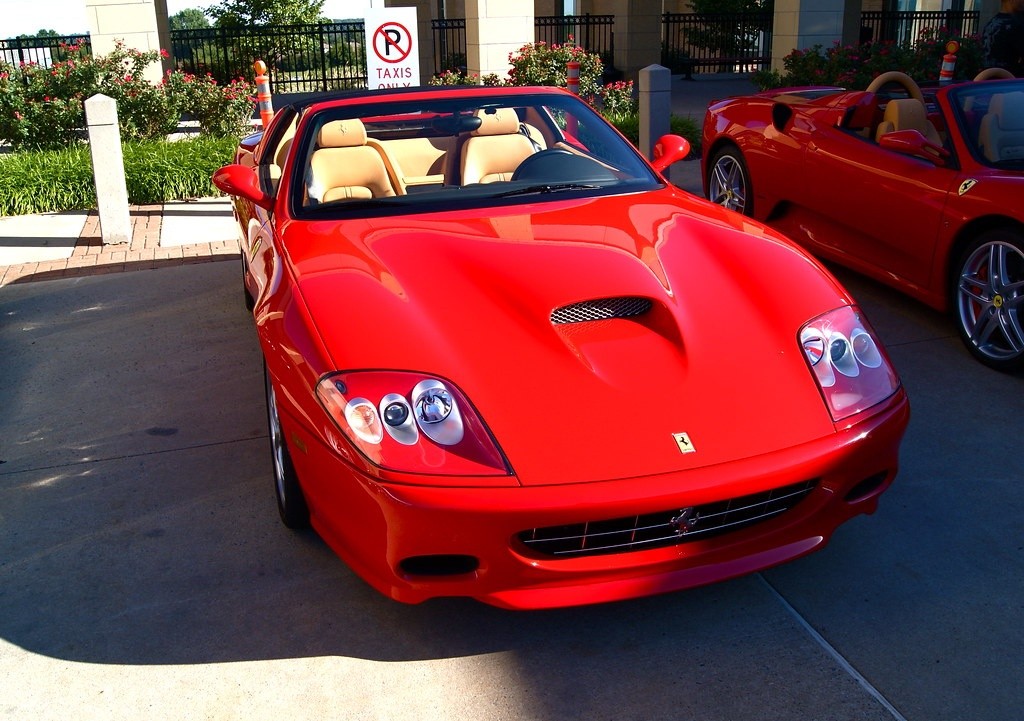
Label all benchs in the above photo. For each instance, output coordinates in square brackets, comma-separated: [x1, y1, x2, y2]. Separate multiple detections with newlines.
[372, 123, 549, 186]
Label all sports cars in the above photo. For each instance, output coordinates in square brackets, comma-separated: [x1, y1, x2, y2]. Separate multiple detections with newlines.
[214, 84, 910, 614]
[699, 69, 1024, 378]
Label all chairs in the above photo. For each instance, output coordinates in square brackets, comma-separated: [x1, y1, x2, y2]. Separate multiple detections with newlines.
[979, 90, 1024, 162]
[305, 118, 408, 204]
[460, 107, 535, 185]
[875, 99, 943, 158]
